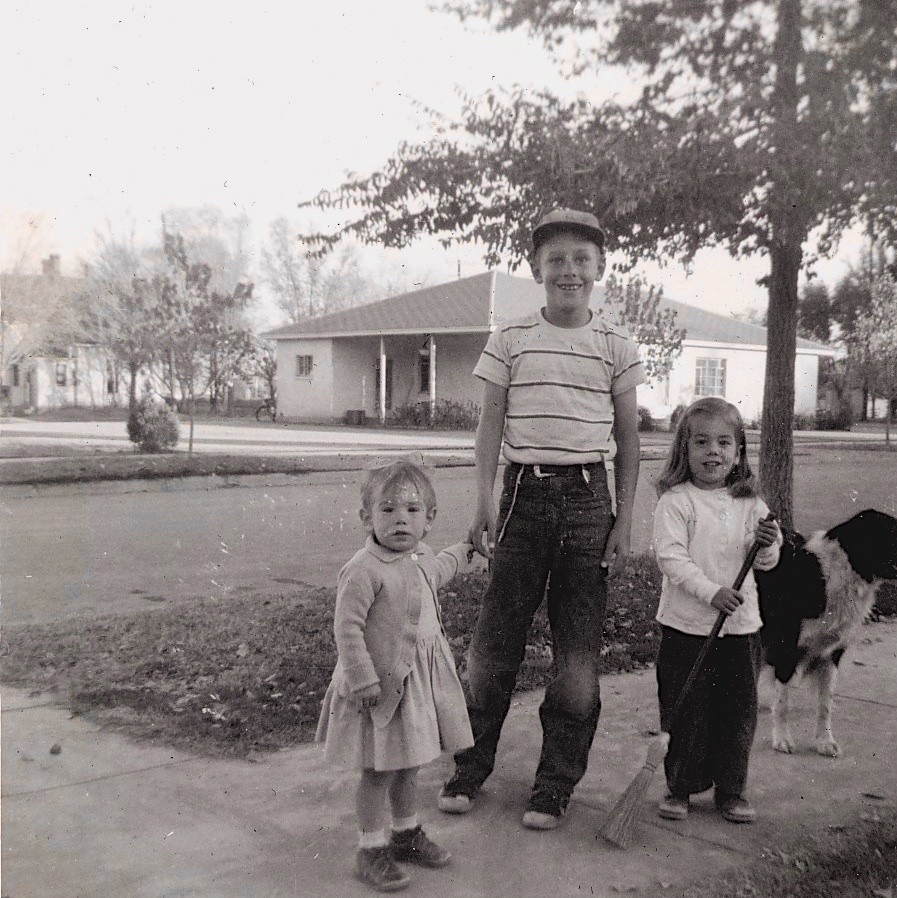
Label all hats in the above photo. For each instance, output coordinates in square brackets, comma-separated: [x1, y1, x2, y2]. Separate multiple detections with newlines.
[532, 208, 607, 252]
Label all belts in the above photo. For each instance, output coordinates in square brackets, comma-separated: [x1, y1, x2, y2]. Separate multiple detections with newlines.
[521, 462, 583, 478]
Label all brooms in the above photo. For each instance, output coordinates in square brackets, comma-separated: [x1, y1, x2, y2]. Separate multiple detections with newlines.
[598, 511, 777, 850]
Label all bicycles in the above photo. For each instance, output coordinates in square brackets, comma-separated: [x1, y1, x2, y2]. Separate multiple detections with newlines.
[255, 397, 277, 423]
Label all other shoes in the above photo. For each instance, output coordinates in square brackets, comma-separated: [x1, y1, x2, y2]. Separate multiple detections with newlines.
[713, 787, 757, 823]
[657, 789, 689, 819]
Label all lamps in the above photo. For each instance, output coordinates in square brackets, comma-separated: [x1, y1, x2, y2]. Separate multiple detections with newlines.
[418, 338, 430, 356]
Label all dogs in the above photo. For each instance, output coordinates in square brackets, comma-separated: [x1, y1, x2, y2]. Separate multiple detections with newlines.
[753, 509, 897, 758]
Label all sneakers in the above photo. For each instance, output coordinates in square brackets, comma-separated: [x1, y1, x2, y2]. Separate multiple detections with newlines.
[435, 762, 482, 811]
[356, 843, 410, 891]
[388, 826, 452, 866]
[524, 783, 571, 830]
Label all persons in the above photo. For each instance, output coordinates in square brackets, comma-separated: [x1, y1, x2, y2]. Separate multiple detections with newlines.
[650, 398, 782, 824]
[314, 461, 476, 888]
[437, 207, 640, 828]
